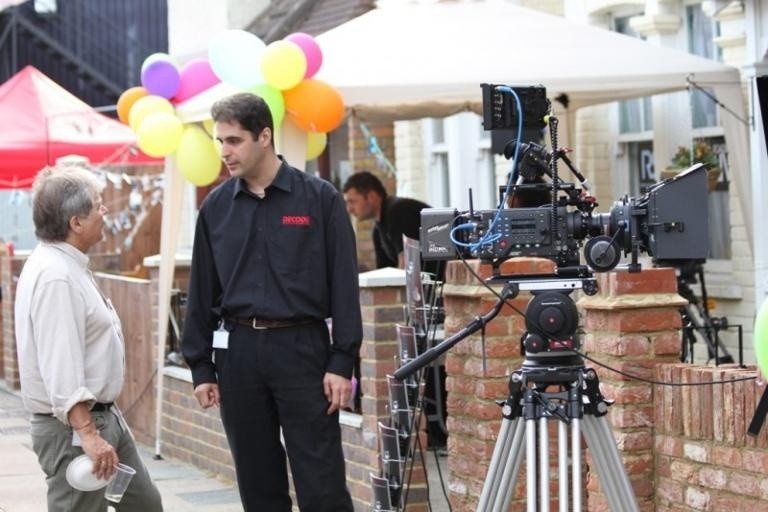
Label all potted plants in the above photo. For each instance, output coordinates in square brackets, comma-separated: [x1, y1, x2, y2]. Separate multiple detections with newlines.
[660, 142, 721, 192]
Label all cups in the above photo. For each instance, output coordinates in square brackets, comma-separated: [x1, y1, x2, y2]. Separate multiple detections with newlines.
[103, 462, 136, 504]
[5, 243, 16, 257]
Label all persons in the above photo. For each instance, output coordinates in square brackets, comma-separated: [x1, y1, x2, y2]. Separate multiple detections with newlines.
[343, 172, 447, 457]
[14, 166, 163, 512]
[180, 92, 363, 511]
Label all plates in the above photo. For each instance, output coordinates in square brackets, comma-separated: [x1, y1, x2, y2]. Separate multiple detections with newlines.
[64, 454, 112, 493]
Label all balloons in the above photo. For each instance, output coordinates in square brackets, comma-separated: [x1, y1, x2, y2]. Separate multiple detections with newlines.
[116, 30, 344, 187]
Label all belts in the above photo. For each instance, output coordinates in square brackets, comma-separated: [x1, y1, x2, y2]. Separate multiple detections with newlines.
[228, 318, 312, 328]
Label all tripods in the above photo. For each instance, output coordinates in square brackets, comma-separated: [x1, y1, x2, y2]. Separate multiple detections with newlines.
[476, 368, 641, 512]
